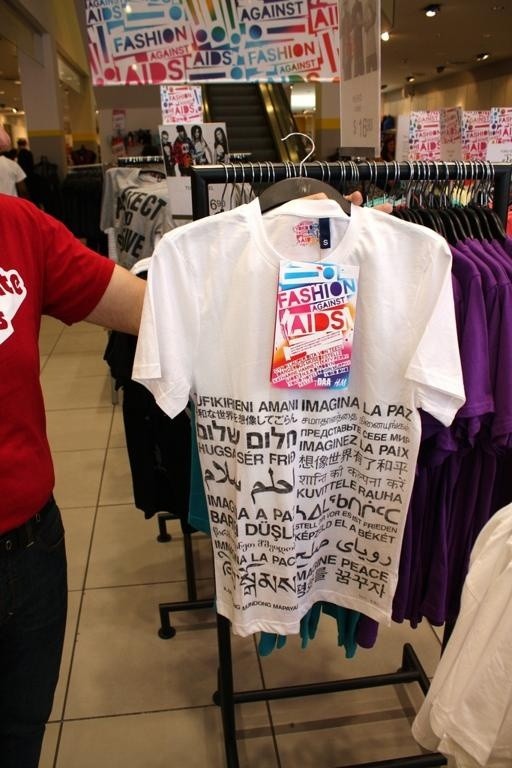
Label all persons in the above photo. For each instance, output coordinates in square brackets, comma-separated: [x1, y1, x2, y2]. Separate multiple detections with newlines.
[17, 138, 35, 193]
[214, 127, 228, 163]
[161, 130, 178, 176]
[0, 189, 395, 768]
[380, 137, 396, 162]
[141, 131, 160, 156]
[1, 124, 28, 200]
[75, 145, 91, 172]
[32, 155, 57, 204]
[173, 125, 197, 176]
[190, 125, 213, 164]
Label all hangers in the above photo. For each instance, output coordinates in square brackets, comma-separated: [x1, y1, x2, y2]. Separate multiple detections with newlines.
[188, 131, 510, 283]
